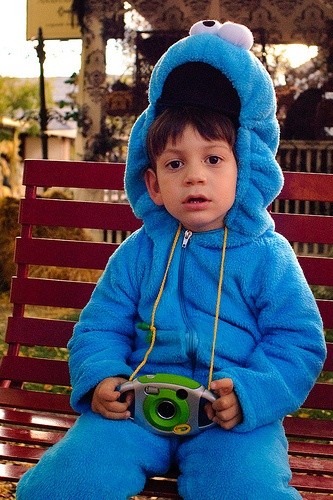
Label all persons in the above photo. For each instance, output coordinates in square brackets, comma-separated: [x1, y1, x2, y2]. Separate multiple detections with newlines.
[284, 88, 328, 141]
[15, 96, 327, 499]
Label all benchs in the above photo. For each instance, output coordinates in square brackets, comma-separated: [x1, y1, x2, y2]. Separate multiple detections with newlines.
[0, 159, 333, 500]
